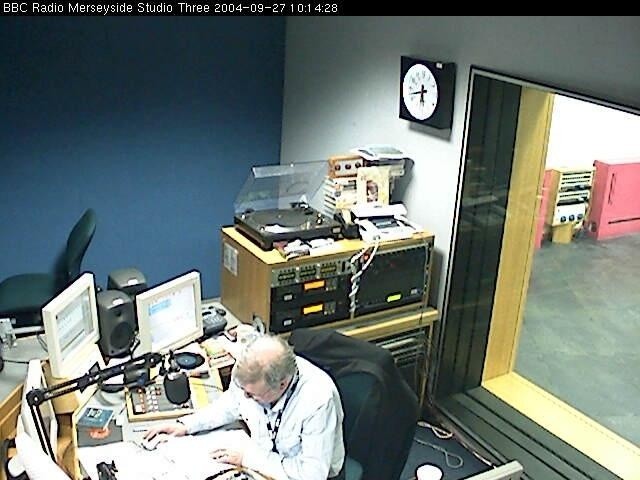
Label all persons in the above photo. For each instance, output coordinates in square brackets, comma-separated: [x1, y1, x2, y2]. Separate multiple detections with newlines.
[142, 333, 348, 480]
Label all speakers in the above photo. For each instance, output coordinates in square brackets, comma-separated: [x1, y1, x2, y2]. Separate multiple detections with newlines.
[96, 290, 136, 356]
[108, 269, 147, 331]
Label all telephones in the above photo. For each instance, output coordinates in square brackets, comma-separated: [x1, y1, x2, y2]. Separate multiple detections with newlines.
[201, 309, 227, 335]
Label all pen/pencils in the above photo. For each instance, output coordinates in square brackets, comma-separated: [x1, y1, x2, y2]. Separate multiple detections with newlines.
[133, 430, 148, 432]
[120, 406, 126, 414]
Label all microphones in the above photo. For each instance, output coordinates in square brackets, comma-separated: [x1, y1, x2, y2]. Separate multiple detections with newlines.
[164, 366, 191, 405]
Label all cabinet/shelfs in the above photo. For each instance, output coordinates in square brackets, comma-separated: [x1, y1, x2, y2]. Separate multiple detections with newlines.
[544, 164, 597, 227]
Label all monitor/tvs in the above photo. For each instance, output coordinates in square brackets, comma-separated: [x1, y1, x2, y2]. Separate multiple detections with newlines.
[135, 268, 204, 364]
[42, 272, 100, 381]
[8, 359, 71, 480]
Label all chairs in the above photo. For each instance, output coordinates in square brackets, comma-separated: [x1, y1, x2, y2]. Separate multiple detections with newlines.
[0, 208, 97, 371]
[287, 326, 395, 480]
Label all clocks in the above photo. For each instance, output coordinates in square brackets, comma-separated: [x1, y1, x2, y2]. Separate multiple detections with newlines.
[398, 55, 457, 129]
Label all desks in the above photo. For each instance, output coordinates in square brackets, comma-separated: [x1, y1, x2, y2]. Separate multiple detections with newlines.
[1, 301, 441, 479]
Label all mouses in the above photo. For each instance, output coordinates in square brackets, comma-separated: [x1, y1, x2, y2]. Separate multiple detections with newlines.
[141, 433, 169, 450]
